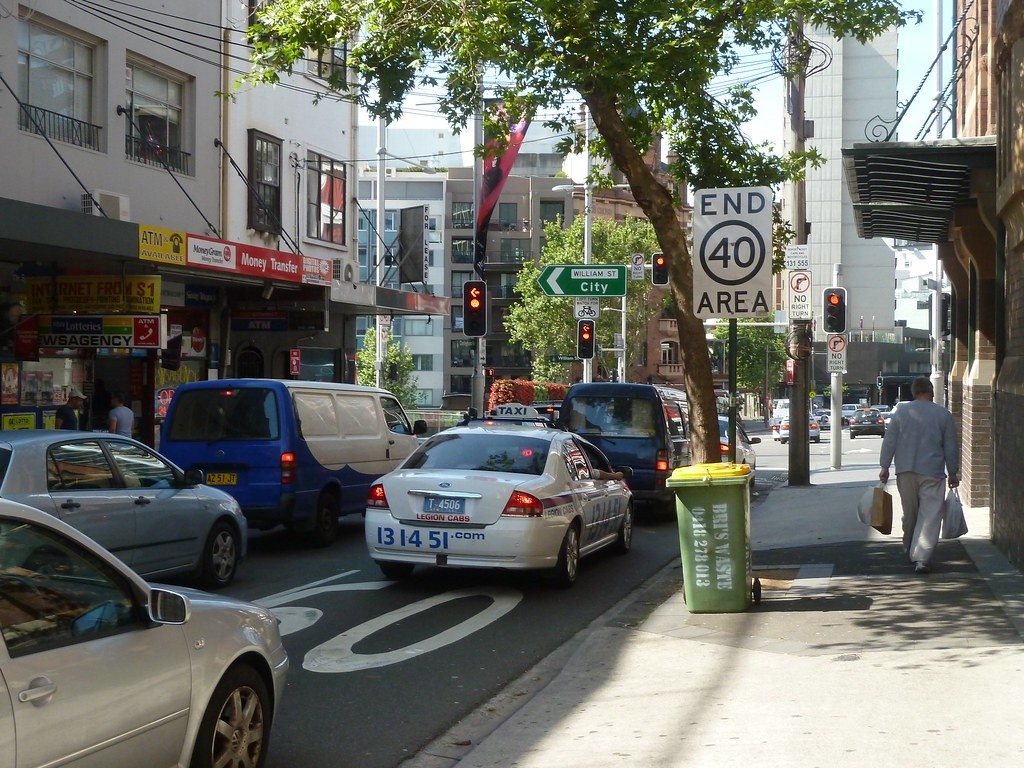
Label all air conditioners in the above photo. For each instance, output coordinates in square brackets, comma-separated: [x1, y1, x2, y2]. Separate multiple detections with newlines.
[82, 188, 129, 222]
[330, 257, 361, 281]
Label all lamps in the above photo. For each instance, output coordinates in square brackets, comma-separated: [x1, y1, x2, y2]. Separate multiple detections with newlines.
[263, 280, 275, 299]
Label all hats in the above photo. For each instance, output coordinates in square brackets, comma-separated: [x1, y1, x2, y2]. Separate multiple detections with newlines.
[68, 389, 88, 400]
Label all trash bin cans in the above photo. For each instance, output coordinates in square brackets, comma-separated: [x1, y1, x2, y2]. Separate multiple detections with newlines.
[666, 462, 761, 613]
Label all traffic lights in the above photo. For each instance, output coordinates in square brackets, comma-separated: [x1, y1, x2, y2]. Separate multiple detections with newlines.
[577, 317, 595, 360]
[651, 252, 670, 286]
[462, 280, 488, 338]
[877, 376, 883, 387]
[822, 287, 846, 335]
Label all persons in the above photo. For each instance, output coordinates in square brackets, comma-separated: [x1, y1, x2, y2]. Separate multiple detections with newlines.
[55, 389, 88, 431]
[107, 392, 134, 439]
[879, 376, 960, 572]
[593, 469, 624, 481]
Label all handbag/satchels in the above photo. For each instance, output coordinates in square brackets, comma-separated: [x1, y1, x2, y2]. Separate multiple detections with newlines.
[942, 486, 968, 539]
[869, 480, 892, 535]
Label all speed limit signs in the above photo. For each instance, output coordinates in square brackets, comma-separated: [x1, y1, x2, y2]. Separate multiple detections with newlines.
[693, 187, 771, 317]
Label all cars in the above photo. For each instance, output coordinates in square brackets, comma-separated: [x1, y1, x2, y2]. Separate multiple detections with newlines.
[364, 403, 635, 590]
[848, 408, 888, 439]
[717, 415, 761, 486]
[0, 427, 249, 589]
[1, 497, 290, 768]
[769, 398, 911, 444]
[528, 400, 563, 430]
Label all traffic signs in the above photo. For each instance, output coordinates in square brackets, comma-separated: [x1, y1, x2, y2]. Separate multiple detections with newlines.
[537, 263, 627, 297]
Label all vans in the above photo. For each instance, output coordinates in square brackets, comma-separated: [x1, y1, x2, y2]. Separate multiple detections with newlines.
[556, 382, 691, 525]
[841, 404, 862, 425]
[157, 378, 427, 549]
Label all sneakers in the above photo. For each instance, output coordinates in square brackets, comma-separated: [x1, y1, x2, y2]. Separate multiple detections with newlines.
[914, 561, 930, 574]
